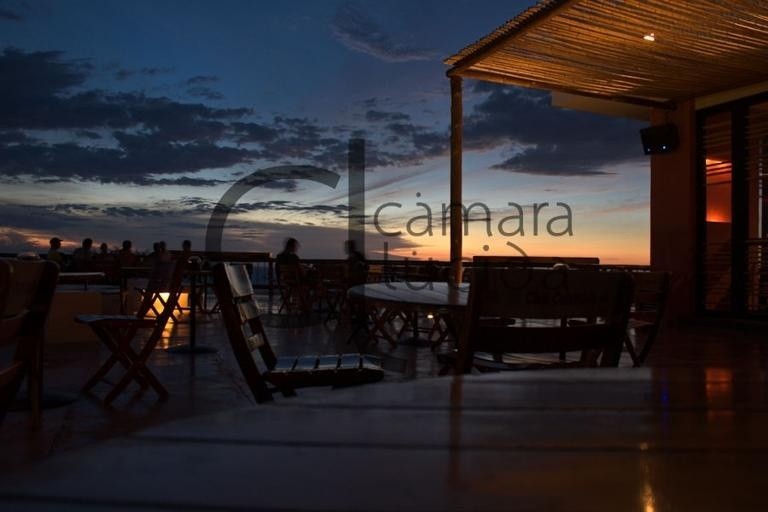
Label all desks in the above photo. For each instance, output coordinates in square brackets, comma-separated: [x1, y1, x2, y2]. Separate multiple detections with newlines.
[118, 266, 221, 319]
[345, 280, 474, 375]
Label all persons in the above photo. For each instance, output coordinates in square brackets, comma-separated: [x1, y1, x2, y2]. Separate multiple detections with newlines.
[47, 237, 202, 284]
[275, 237, 328, 316]
[336, 238, 370, 322]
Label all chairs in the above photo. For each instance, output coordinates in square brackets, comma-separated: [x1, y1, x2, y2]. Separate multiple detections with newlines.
[0, 256, 193, 432]
[447, 264, 674, 372]
[216, 258, 384, 404]
